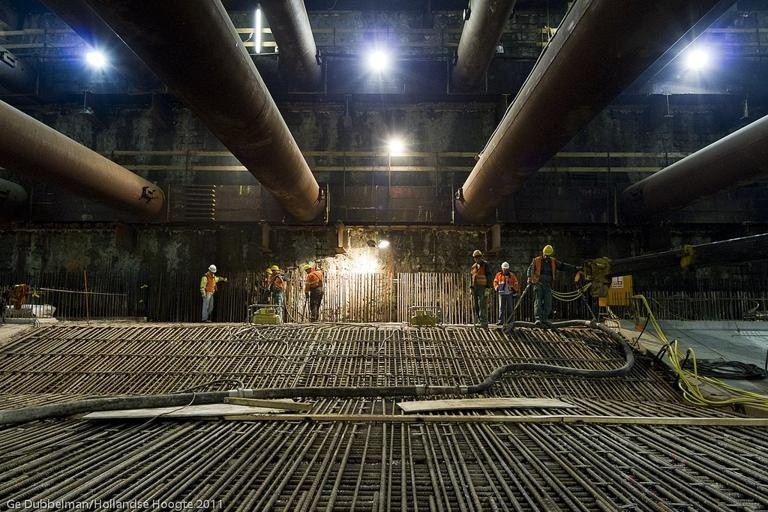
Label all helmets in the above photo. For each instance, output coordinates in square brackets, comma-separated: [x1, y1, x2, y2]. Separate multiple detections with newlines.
[208, 265, 216, 272]
[543, 245, 553, 255]
[265, 265, 279, 273]
[472, 250, 482, 257]
[304, 265, 311, 270]
[501, 262, 509, 269]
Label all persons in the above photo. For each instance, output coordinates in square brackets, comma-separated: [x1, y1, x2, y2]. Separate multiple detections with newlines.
[526, 243, 583, 324]
[268, 265, 291, 306]
[303, 265, 324, 321]
[493, 261, 520, 324]
[257, 267, 273, 304]
[574, 269, 585, 319]
[469, 249, 493, 327]
[199, 265, 229, 323]
[11, 285, 36, 311]
